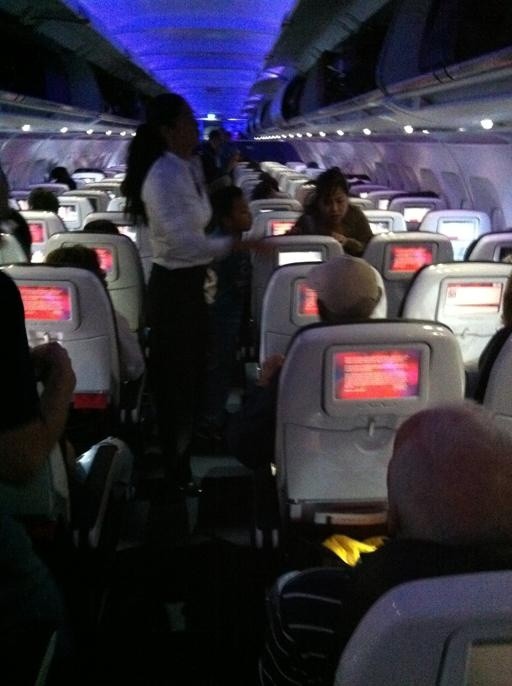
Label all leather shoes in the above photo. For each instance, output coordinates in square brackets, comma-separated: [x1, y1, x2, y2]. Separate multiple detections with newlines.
[161, 465, 205, 498]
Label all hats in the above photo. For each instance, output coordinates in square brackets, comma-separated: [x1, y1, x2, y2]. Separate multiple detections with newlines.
[387, 405, 511, 542]
[306, 257, 380, 314]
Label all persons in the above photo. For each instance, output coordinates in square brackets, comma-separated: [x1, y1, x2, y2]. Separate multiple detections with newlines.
[2, 93, 511, 499]
[254, 401, 512, 686]
